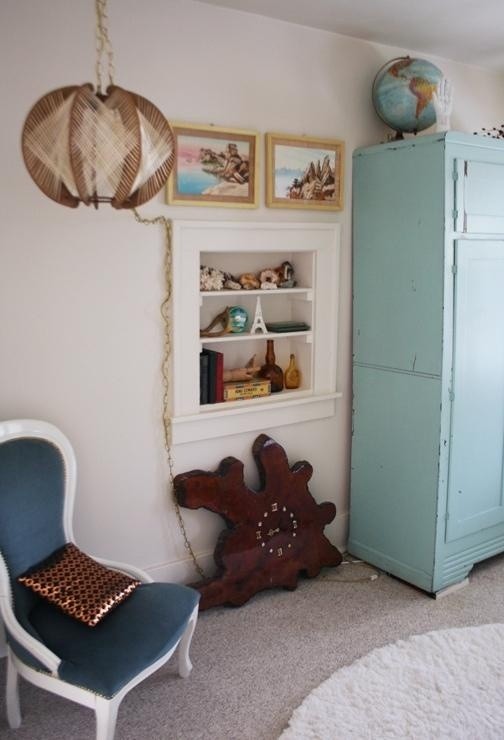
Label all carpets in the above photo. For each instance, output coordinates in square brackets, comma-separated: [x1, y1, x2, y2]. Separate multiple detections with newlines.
[268, 618, 504, 738]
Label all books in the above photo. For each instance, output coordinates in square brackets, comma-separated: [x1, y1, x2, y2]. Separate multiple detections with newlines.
[200, 347, 272, 405]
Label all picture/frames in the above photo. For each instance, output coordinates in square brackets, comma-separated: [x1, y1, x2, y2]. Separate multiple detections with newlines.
[262, 130, 347, 215]
[160, 118, 261, 210]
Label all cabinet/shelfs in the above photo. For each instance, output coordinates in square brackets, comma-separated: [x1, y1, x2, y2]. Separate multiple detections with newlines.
[343, 123, 504, 601]
[159, 215, 345, 450]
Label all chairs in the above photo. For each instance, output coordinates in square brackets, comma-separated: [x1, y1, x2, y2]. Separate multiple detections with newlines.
[0, 416, 203, 740]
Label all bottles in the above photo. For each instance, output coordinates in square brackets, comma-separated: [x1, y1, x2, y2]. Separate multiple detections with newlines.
[258, 341, 282, 393]
[285, 353, 300, 387]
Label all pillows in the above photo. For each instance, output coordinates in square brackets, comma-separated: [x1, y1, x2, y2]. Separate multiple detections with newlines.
[14, 538, 141, 631]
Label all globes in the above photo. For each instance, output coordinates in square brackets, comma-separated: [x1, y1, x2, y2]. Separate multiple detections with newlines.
[371, 56, 449, 142]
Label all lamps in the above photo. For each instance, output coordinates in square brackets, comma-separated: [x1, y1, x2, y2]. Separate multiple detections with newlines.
[19, 0, 176, 215]
